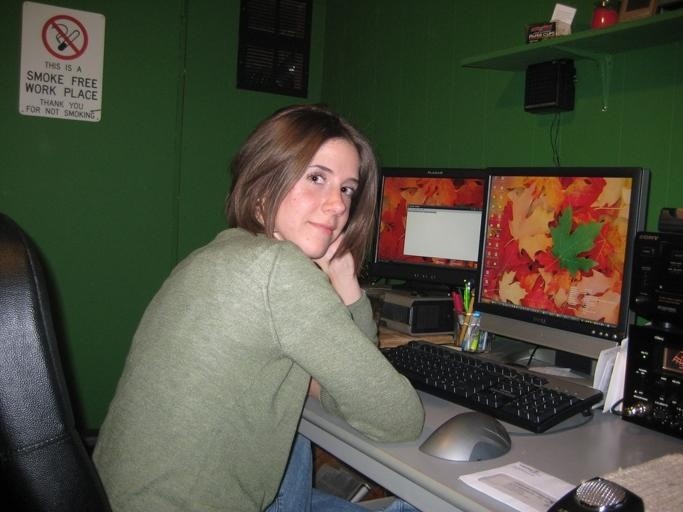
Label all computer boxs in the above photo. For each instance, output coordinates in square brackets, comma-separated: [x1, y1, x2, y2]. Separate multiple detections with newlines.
[363, 284, 460, 337]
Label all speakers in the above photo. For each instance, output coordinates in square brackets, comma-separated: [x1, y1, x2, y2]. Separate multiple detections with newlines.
[524, 60, 576, 113]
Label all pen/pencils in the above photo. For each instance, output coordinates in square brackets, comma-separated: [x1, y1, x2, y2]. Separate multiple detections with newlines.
[451, 280, 475, 352]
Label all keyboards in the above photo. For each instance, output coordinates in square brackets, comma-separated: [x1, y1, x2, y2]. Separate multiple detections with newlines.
[380, 336, 605, 437]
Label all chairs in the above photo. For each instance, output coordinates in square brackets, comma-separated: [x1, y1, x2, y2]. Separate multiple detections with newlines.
[0, 213, 120, 511]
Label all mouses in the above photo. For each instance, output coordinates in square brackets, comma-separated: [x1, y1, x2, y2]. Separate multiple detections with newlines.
[419, 408, 513, 462]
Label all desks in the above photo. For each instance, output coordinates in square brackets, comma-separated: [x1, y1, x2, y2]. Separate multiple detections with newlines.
[298, 327, 683, 512]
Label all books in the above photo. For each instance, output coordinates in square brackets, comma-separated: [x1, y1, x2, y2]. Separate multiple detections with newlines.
[588, 336, 630, 415]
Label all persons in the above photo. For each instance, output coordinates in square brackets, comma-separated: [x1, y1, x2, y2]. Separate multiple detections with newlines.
[90, 103, 424, 512]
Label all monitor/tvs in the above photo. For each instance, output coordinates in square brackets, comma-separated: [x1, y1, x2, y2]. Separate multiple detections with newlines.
[474, 164, 651, 390]
[367, 165, 487, 295]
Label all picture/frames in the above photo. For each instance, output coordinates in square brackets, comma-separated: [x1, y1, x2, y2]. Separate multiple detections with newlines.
[618, 0, 657, 20]
